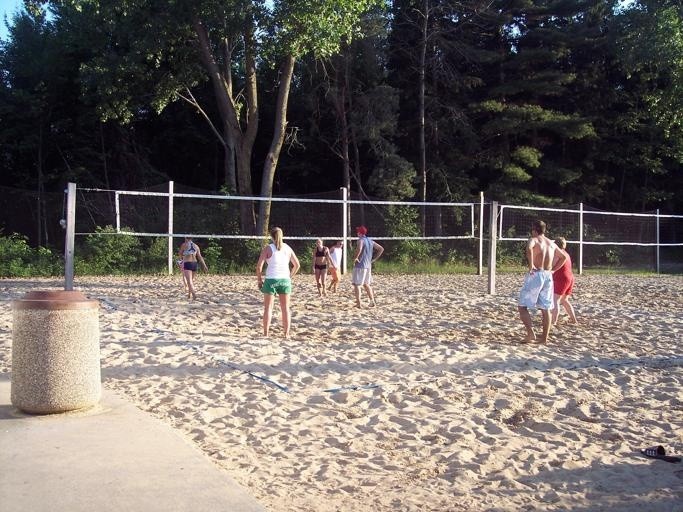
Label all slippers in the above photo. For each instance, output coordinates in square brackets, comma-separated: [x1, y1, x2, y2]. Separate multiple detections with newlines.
[641, 445, 681, 463]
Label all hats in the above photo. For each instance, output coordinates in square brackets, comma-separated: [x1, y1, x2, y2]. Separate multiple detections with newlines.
[357, 225, 367, 234]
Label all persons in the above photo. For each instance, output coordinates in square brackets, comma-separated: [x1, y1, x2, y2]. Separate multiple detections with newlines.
[327, 239, 343, 293]
[312, 238, 337, 297]
[549, 237, 578, 325]
[353, 225, 384, 307]
[517, 220, 566, 345]
[177, 242, 189, 291]
[179, 235, 208, 300]
[256, 227, 300, 340]
